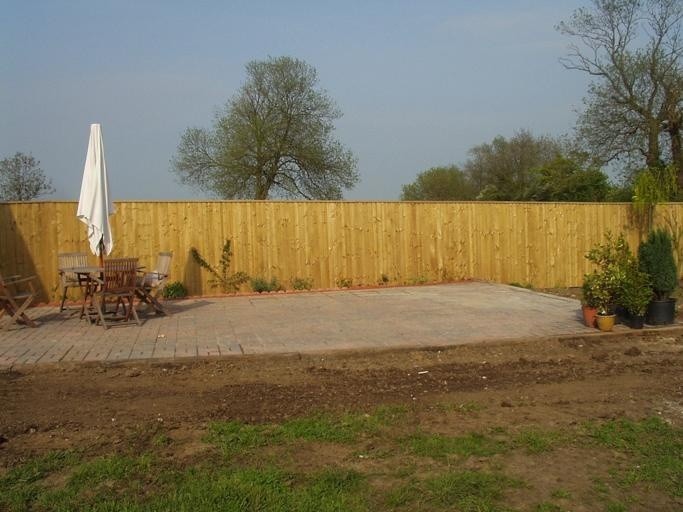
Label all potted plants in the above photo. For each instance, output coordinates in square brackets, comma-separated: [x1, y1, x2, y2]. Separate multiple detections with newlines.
[581, 227, 677, 330]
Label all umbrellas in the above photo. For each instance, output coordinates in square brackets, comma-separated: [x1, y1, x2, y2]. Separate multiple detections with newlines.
[73, 121, 116, 312]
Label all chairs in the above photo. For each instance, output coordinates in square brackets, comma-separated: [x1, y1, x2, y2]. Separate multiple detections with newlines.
[56, 253, 173, 330]
[0, 269, 38, 331]
